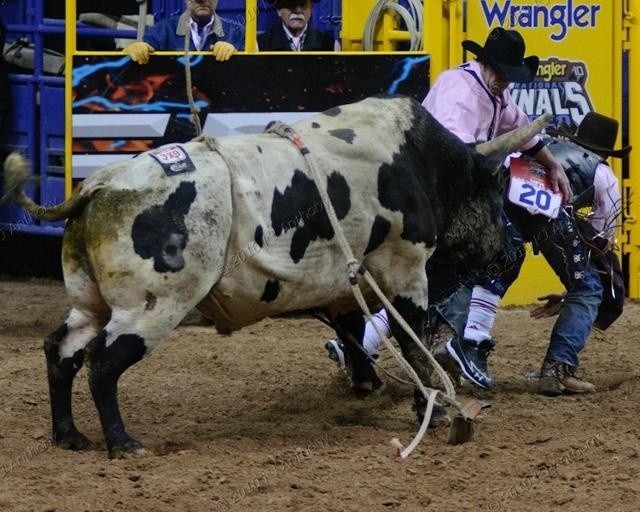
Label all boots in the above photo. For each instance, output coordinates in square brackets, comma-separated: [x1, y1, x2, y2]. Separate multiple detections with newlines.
[537, 359, 594, 394]
[431, 322, 463, 388]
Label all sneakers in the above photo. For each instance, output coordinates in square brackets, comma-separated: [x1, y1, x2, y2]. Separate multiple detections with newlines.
[324, 338, 378, 388]
[445, 320, 494, 390]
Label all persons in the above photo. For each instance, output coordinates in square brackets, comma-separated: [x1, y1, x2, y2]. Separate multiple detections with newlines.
[121, 0, 245, 64]
[323, 26, 573, 399]
[257, 0, 340, 52]
[429, 113, 633, 396]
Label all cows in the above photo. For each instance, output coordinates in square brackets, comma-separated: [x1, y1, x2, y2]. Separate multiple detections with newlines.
[0, 93, 552, 460]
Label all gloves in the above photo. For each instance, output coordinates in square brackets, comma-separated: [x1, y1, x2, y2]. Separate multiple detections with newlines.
[210, 41, 236, 63]
[123, 42, 155, 65]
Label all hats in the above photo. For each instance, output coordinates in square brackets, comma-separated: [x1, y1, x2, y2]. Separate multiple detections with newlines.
[461, 27, 539, 84]
[559, 112, 632, 158]
